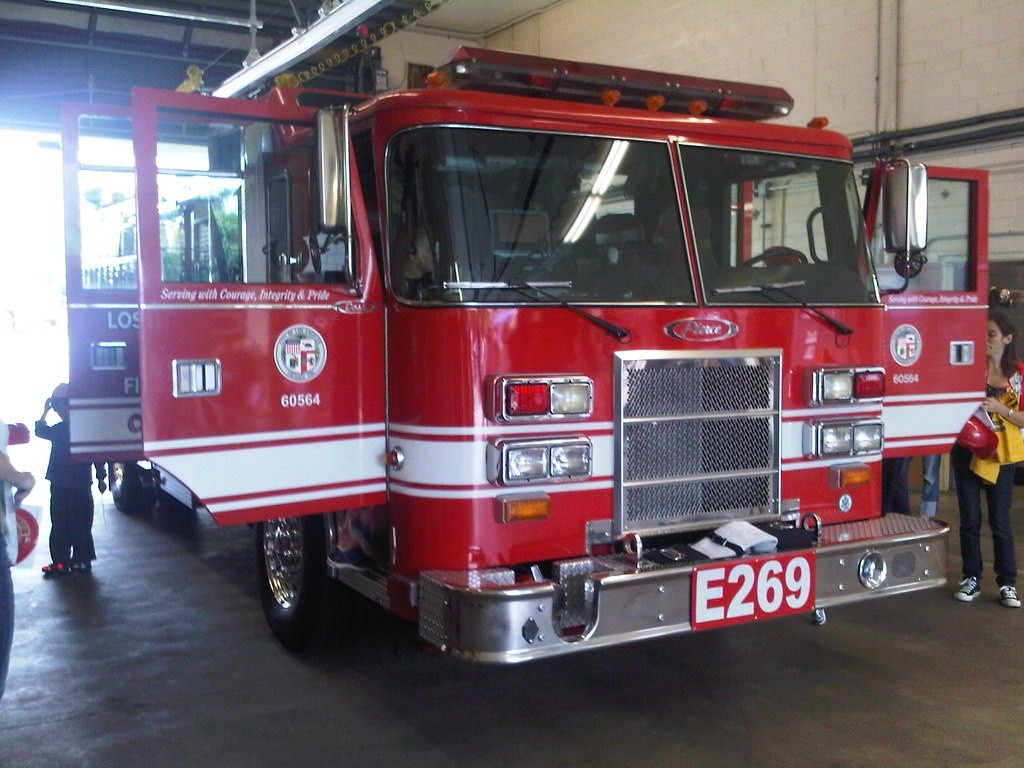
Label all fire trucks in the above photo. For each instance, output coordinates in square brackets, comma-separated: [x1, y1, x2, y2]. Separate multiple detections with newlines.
[43, 44, 994, 688]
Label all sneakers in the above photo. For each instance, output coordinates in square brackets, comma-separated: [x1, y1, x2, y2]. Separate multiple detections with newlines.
[954, 577, 981, 601]
[997, 586, 1021, 607]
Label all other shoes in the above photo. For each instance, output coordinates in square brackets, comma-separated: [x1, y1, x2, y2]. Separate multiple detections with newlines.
[325, 544, 386, 577]
[350, 517, 391, 574]
[43, 562, 70, 573]
[69, 561, 92, 573]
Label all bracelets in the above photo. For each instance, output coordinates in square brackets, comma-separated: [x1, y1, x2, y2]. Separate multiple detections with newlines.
[1003, 408, 1013, 420]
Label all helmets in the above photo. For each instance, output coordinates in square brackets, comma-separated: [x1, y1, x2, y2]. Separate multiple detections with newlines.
[958, 415, 998, 461]
[10, 507, 39, 565]
[50, 383, 69, 399]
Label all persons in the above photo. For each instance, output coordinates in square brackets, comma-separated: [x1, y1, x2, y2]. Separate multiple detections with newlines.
[892, 454, 941, 517]
[34, 383, 108, 574]
[953, 314, 1024, 607]
[0, 420, 34, 700]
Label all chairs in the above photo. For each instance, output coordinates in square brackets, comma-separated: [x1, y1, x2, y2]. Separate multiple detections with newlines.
[651, 201, 720, 303]
